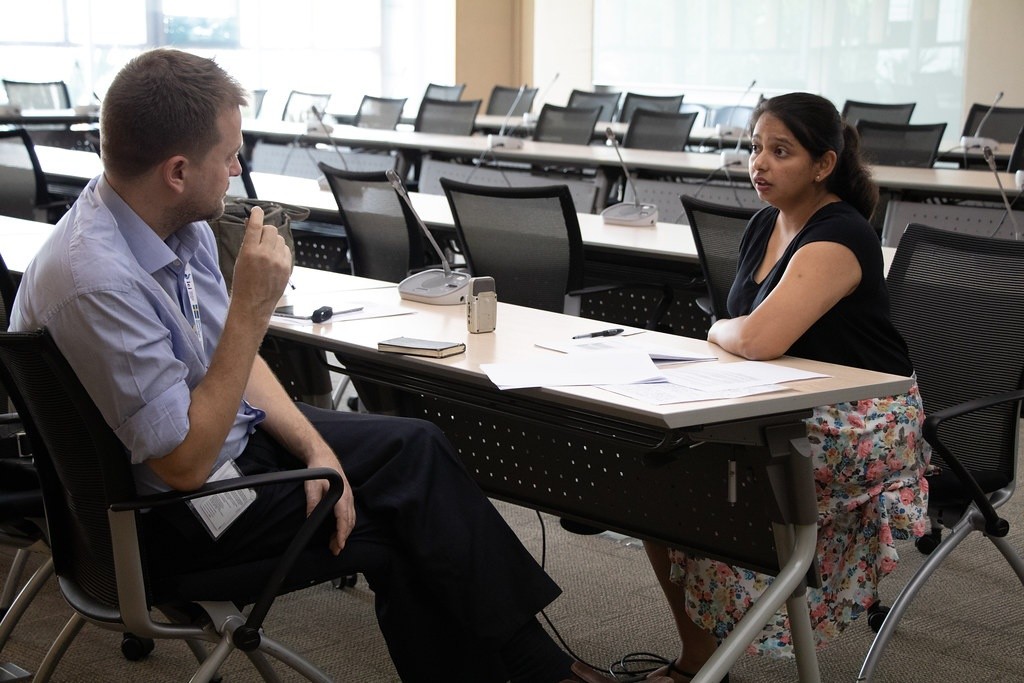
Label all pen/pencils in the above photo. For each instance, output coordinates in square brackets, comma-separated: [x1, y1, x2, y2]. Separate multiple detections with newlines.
[244, 206, 296, 290]
[572, 328, 624, 340]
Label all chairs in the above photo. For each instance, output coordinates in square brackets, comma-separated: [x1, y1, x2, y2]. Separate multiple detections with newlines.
[0, 128, 59, 224]
[318, 161, 426, 284]
[254, 85, 1024, 178]
[226, 151, 257, 199]
[0, 348, 344, 683]
[855, 223, 1024, 683]
[680, 195, 759, 320]
[0, 253, 52, 622]
[439, 176, 674, 331]
[3, 79, 72, 109]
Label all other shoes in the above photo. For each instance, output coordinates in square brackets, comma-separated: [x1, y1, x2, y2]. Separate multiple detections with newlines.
[622, 658, 729, 683]
[560, 518, 605, 535]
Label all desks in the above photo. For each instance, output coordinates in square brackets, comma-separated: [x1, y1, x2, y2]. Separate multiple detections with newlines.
[0, 104, 1024, 683]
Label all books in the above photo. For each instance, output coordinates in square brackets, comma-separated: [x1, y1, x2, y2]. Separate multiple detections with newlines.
[377, 337, 466, 359]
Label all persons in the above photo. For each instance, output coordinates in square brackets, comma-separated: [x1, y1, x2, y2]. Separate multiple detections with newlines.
[646, 92, 932, 683]
[7, 48, 610, 682]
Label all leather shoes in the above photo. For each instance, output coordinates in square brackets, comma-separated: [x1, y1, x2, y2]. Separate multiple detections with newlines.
[558, 660, 672, 683]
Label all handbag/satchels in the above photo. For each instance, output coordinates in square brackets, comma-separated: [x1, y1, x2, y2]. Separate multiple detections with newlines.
[0, 412, 40, 489]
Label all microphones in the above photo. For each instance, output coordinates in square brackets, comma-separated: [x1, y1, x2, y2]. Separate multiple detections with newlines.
[92, 92, 103, 104]
[931, 91, 1024, 242]
[310, 72, 765, 305]
